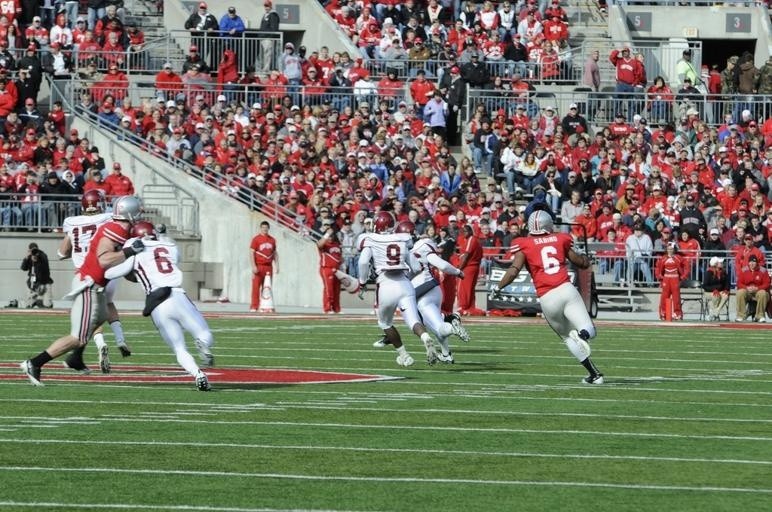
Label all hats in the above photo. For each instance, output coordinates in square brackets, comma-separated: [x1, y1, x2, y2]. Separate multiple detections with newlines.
[190, 46, 197, 51]
[388, 0, 394, 5]
[298, 142, 306, 146]
[403, 124, 410, 130]
[658, 131, 665, 137]
[104, 102, 112, 107]
[128, 22, 136, 26]
[238, 156, 246, 161]
[157, 97, 164, 103]
[487, 177, 495, 185]
[512, 34, 520, 37]
[445, 42, 450, 47]
[114, 163, 120, 169]
[318, 174, 324, 180]
[308, 67, 316, 73]
[739, 206, 747, 211]
[71, 129, 77, 135]
[685, 179, 692, 184]
[417, 200, 423, 206]
[620, 166, 628, 170]
[710, 256, 724, 267]
[21, 163, 28, 169]
[467, 193, 475, 200]
[768, 208, 772, 213]
[730, 125, 736, 129]
[720, 168, 728, 173]
[360, 102, 368, 108]
[528, 11, 534, 16]
[285, 42, 294, 49]
[309, 146, 315, 150]
[749, 254, 757, 262]
[451, 66, 459, 73]
[653, 185, 660, 191]
[28, 45, 35, 51]
[25, 98, 34, 105]
[607, 134, 614, 140]
[226, 167, 234, 173]
[216, 296, 231, 304]
[335, 67, 344, 73]
[552, 0, 558, 3]
[766, 152, 772, 159]
[229, 7, 235, 13]
[744, 234, 752, 240]
[723, 158, 730, 163]
[260, 165, 266, 169]
[667, 196, 674, 202]
[196, 95, 204, 100]
[264, 0, 272, 6]
[494, 194, 502, 202]
[686, 107, 698, 116]
[418, 182, 425, 187]
[640, 118, 646, 123]
[403, 115, 411, 121]
[288, 192, 299, 199]
[629, 172, 636, 177]
[433, 30, 440, 35]
[355, 189, 362, 193]
[527, 0, 536, 4]
[324, 100, 331, 105]
[363, 217, 371, 223]
[570, 103, 577, 109]
[727, 59, 737, 64]
[0, 68, 7, 74]
[388, 27, 395, 33]
[684, 77, 690, 81]
[289, 126, 296, 132]
[340, 115, 348, 120]
[629, 205, 636, 209]
[347, 152, 356, 157]
[275, 104, 282, 110]
[253, 102, 262, 109]
[33, 16, 41, 22]
[719, 147, 728, 152]
[290, 159, 298, 164]
[626, 184, 635, 189]
[88, 60, 97, 65]
[277, 135, 284, 140]
[441, 200, 449, 206]
[467, 30, 473, 35]
[632, 194, 639, 199]
[286, 118, 294, 125]
[568, 171, 576, 177]
[512, 74, 520, 83]
[328, 116, 336, 123]
[423, 122, 430, 128]
[267, 113, 274, 119]
[319, 127, 326, 131]
[471, 52, 478, 57]
[321, 207, 328, 212]
[262, 103, 269, 109]
[27, 128, 35, 135]
[165, 100, 176, 108]
[366, 184, 373, 190]
[205, 115, 213, 121]
[299, 46, 305, 53]
[422, 157, 430, 162]
[490, 203, 497, 211]
[200, 2, 207, 9]
[122, 115, 132, 123]
[351, 166, 356, 172]
[359, 139, 368, 147]
[29, 243, 37, 249]
[163, 63, 171, 69]
[229, 151, 237, 157]
[751, 183, 759, 191]
[663, 227, 672, 233]
[742, 110, 751, 117]
[248, 172, 256, 179]
[546, 106, 553, 111]
[658, 119, 666, 125]
[253, 128, 262, 136]
[633, 224, 642, 230]
[358, 152, 366, 158]
[49, 42, 59, 49]
[401, 159, 407, 164]
[272, 173, 279, 179]
[153, 123, 165, 130]
[596, 189, 602, 194]
[375, 108, 382, 114]
[398, 101, 406, 107]
[519, 206, 527, 212]
[227, 130, 235, 135]
[395, 134, 403, 140]
[516, 104, 523, 109]
[339, 150, 344, 155]
[414, 37, 422, 44]
[615, 112, 623, 117]
[482, 207, 488, 214]
[448, 215, 456, 222]
[545, 128, 551, 136]
[217, 95, 226, 102]
[196, 123, 204, 129]
[344, 218, 352, 225]
[385, 17, 392, 24]
[47, 172, 56, 178]
[749, 121, 756, 126]
[538, 33, 545, 39]
[480, 34, 488, 39]
[256, 175, 265, 182]
[705, 184, 711, 189]
[0, 39, 8, 47]
[90, 147, 98, 153]
[722, 179, 732, 186]
[155, 142, 166, 149]
[710, 228, 719, 235]
[172, 128, 181, 134]
[672, 159, 680, 164]
[683, 51, 690, 55]
[322, 219, 330, 226]
[387, 185, 394, 190]
[392, 36, 399, 41]
[9, 127, 17, 134]
[290, 105, 300, 112]
[366, 147, 375, 152]
[667, 152, 675, 157]
[613, 213, 621, 219]
[553, 10, 559, 17]
[508, 201, 514, 204]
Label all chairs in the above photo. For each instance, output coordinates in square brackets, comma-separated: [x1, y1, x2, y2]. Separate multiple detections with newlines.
[492, 84, 645, 120]
[128, 78, 158, 104]
[676, 279, 772, 322]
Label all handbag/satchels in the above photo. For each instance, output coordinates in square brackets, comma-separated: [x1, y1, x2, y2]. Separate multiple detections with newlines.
[695, 78, 701, 84]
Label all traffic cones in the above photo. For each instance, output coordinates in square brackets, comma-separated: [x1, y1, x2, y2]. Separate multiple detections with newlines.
[259, 271, 275, 314]
[331, 267, 361, 294]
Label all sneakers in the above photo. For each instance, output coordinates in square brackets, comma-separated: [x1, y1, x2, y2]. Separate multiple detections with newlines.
[662, 313, 666, 320]
[196, 369, 212, 391]
[192, 337, 214, 368]
[323, 310, 335, 314]
[444, 312, 460, 324]
[99, 343, 110, 374]
[569, 329, 591, 358]
[117, 342, 130, 357]
[372, 337, 385, 347]
[600, 6, 605, 12]
[675, 315, 683, 320]
[735, 317, 745, 322]
[582, 373, 603, 385]
[396, 354, 414, 366]
[250, 309, 256, 312]
[424, 337, 437, 366]
[62, 360, 92, 373]
[336, 312, 343, 314]
[434, 350, 454, 364]
[756, 318, 766, 323]
[451, 318, 470, 342]
[19, 360, 45, 387]
[383, 336, 390, 344]
[706, 315, 720, 322]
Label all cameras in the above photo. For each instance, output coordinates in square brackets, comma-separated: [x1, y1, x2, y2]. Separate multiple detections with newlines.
[32, 249, 38, 256]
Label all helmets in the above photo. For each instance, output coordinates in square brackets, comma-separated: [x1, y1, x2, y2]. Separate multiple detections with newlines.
[82, 189, 107, 213]
[528, 210, 553, 235]
[129, 221, 155, 239]
[373, 212, 394, 234]
[112, 195, 143, 225]
[395, 221, 415, 235]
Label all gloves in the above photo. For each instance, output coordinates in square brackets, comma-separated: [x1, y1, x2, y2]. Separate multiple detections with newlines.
[712, 288, 720, 297]
[722, 288, 730, 296]
[359, 288, 368, 300]
[122, 236, 144, 258]
[153, 224, 166, 233]
[56, 249, 70, 261]
[458, 270, 464, 279]
[576, 248, 586, 256]
[406, 234, 420, 249]
[491, 289, 500, 299]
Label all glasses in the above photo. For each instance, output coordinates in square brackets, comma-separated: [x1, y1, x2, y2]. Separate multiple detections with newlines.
[66, 176, 71, 177]
[609, 153, 614, 155]
[660, 147, 665, 150]
[363, 116, 369, 119]
[321, 115, 326, 118]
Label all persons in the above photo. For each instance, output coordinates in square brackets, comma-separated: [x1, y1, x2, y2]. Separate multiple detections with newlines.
[397, 221, 471, 365]
[1, 2, 772, 323]
[18, 194, 167, 388]
[56, 188, 133, 374]
[357, 243, 461, 349]
[104, 222, 215, 392]
[490, 208, 608, 386]
[360, 208, 438, 367]
